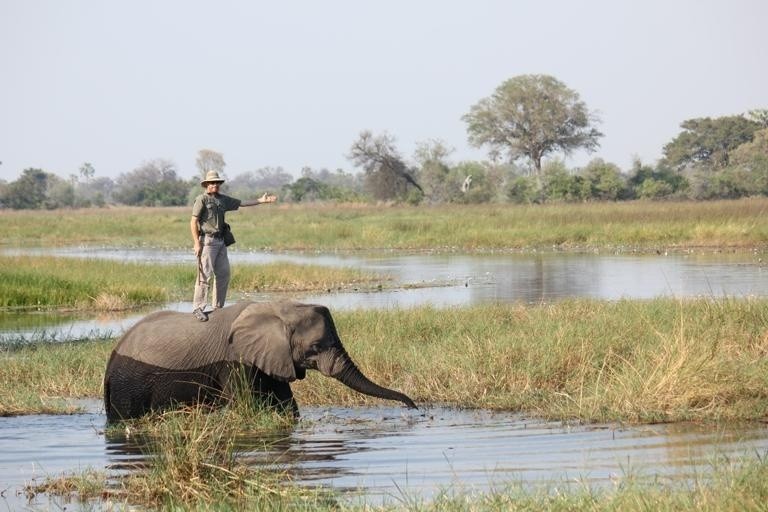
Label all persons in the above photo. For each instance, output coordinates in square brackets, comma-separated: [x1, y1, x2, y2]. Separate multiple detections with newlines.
[190, 170, 277, 322]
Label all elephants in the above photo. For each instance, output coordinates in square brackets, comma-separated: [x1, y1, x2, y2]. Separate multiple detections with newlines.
[103, 299, 419, 428]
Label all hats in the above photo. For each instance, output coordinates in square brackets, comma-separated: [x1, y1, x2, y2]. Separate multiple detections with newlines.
[200, 170, 224, 187]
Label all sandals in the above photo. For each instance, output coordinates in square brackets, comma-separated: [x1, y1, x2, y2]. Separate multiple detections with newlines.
[193, 309, 207, 322]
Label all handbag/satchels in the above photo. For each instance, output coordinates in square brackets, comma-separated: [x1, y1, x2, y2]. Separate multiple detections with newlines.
[223, 223, 235, 246]
